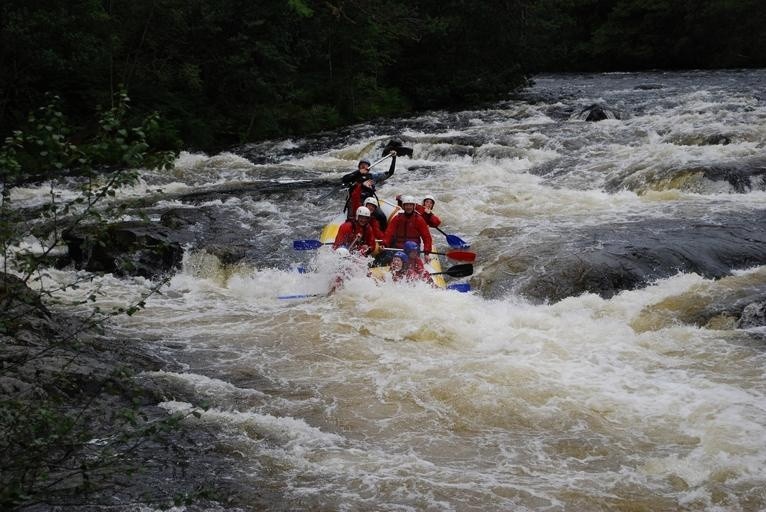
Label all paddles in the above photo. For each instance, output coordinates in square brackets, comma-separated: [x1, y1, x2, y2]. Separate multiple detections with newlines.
[318, 152, 393, 200]
[414, 209, 470, 251]
[429, 264, 474, 277]
[276, 294, 326, 300]
[288, 267, 307, 274]
[383, 247, 476, 261]
[292, 238, 336, 251]
[447, 283, 471, 292]
[378, 198, 396, 207]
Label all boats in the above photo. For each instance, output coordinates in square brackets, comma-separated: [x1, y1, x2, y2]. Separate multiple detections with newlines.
[319, 197, 448, 342]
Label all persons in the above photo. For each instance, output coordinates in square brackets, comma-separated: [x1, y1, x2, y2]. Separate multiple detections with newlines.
[330, 148, 444, 291]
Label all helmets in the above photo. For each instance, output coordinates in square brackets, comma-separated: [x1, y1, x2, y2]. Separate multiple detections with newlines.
[404, 241, 418, 253]
[394, 251, 407, 263]
[356, 206, 370, 221]
[402, 196, 416, 210]
[363, 197, 378, 209]
[358, 159, 370, 167]
[423, 195, 435, 210]
